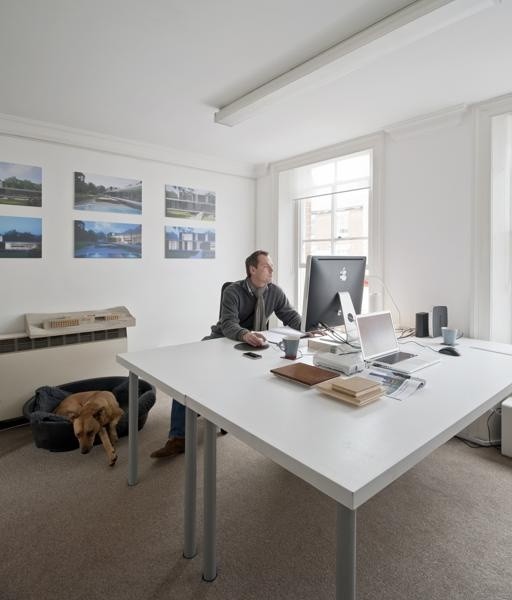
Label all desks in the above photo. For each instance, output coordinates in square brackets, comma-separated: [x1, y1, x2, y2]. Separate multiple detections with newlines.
[117, 312, 396, 559]
[186, 338, 511, 600]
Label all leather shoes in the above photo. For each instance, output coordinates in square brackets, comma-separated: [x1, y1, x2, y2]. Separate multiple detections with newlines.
[150, 439, 181, 459]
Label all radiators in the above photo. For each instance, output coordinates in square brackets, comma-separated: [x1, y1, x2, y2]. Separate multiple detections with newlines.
[1, 327, 128, 430]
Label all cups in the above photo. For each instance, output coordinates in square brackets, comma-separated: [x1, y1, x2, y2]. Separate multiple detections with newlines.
[279, 336, 300, 360]
[441, 326, 465, 345]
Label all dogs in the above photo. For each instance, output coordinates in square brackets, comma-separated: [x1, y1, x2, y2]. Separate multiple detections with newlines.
[54, 390, 125, 468]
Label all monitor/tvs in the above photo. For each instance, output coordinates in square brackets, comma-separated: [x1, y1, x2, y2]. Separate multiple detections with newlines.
[300, 254, 367, 343]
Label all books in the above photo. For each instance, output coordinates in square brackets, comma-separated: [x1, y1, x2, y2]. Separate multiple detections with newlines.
[380, 370, 426, 401]
[317, 376, 387, 407]
[271, 362, 340, 388]
[251, 325, 307, 344]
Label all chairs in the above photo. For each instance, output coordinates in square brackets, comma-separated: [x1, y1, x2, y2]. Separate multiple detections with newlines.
[220, 281, 270, 333]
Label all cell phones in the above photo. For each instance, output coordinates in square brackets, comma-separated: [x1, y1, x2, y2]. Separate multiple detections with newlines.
[243, 351, 263, 360]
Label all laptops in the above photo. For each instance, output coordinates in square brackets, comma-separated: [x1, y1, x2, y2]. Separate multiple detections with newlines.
[354, 309, 437, 374]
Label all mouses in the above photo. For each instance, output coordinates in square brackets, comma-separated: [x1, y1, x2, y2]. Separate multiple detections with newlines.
[257, 337, 270, 348]
[438, 346, 459, 356]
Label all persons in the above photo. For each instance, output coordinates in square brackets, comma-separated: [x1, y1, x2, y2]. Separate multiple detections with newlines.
[150, 249, 327, 458]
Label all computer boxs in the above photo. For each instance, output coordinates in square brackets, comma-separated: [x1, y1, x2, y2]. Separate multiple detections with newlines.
[307, 338, 361, 355]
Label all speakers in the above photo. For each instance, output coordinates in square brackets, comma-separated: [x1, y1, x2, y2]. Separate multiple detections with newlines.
[415, 311, 429, 338]
[432, 304, 449, 337]
